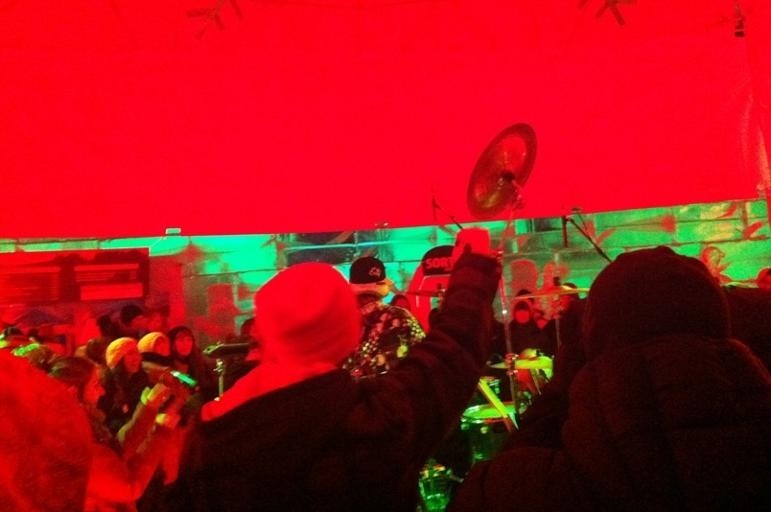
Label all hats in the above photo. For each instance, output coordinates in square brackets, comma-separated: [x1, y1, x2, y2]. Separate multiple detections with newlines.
[349, 257, 389, 296]
[137, 332, 162, 352]
[105, 337, 136, 369]
[583, 246, 730, 341]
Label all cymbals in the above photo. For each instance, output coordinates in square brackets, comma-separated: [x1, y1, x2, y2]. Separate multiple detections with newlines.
[406, 289, 445, 298]
[514, 282, 591, 303]
[487, 355, 554, 370]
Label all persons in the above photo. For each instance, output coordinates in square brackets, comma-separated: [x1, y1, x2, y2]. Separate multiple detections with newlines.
[0, 226, 771, 512]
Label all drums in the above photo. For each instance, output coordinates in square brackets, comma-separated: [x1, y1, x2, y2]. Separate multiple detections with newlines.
[460, 401, 528, 436]
[415, 460, 464, 512]
